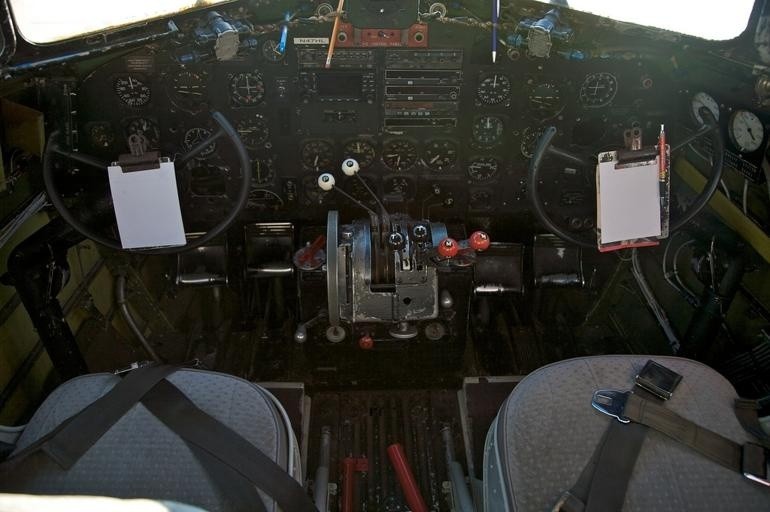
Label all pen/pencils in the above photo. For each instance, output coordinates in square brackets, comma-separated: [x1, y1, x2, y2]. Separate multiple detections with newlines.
[279, 10, 290, 53]
[492, 0, 500, 63]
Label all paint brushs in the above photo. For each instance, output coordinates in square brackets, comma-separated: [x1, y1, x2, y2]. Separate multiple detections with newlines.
[325, 0, 345, 70]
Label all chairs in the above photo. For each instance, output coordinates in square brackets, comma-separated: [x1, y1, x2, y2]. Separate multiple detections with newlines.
[1, 354, 306, 512]
[483, 353, 768, 512]
